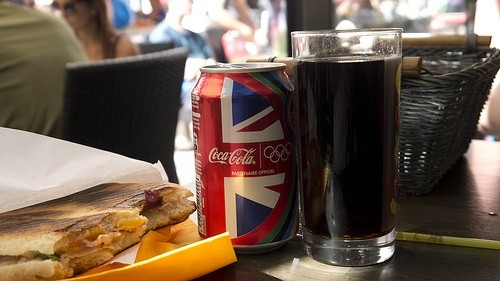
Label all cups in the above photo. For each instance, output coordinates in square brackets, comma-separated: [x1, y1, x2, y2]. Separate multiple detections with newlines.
[292, 29, 402, 266]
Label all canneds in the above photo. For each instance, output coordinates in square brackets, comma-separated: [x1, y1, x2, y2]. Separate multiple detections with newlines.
[191, 62, 299, 254]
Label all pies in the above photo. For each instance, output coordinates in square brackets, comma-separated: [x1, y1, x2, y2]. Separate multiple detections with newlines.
[0, 180, 199, 281]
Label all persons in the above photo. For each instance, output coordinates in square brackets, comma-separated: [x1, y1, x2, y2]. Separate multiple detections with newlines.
[151, 0, 411, 151]
[0, 0, 90, 141]
[53, 0, 139, 62]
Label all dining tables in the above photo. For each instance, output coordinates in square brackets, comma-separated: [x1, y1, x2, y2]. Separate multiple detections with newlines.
[194, 138, 500, 281]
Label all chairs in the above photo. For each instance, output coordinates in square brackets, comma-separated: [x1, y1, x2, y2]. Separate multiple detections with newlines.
[57, 44, 192, 186]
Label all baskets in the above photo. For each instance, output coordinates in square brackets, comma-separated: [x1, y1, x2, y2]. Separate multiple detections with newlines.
[247, 35, 500, 196]
